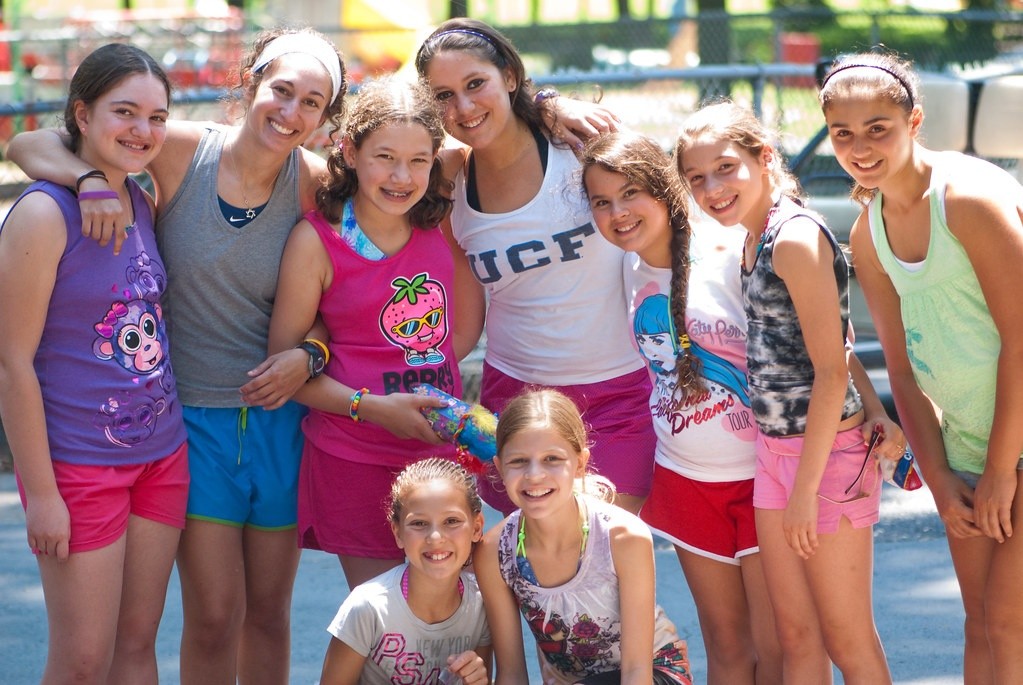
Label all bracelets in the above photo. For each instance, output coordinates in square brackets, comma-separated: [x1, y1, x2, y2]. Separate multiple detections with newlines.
[349, 388, 371, 422]
[75, 170, 119, 200]
[304, 338, 329, 364]
[534, 88, 562, 104]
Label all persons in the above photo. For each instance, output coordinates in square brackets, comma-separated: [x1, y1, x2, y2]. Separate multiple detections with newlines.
[8, 28, 330, 685]
[267, 79, 620, 592]
[675, 102, 893, 685]
[320, 458, 493, 685]
[472, 390, 693, 685]
[0, 41, 187, 685]
[819, 55, 1023, 685]
[581, 130, 906, 685]
[416, 18, 659, 519]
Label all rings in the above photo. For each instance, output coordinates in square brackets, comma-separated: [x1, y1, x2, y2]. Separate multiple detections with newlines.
[897, 445, 903, 453]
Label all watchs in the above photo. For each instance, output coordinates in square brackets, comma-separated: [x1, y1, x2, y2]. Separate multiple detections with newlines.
[293, 342, 325, 383]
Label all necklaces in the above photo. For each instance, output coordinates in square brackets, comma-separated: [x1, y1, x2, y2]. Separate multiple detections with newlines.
[228, 142, 282, 219]
